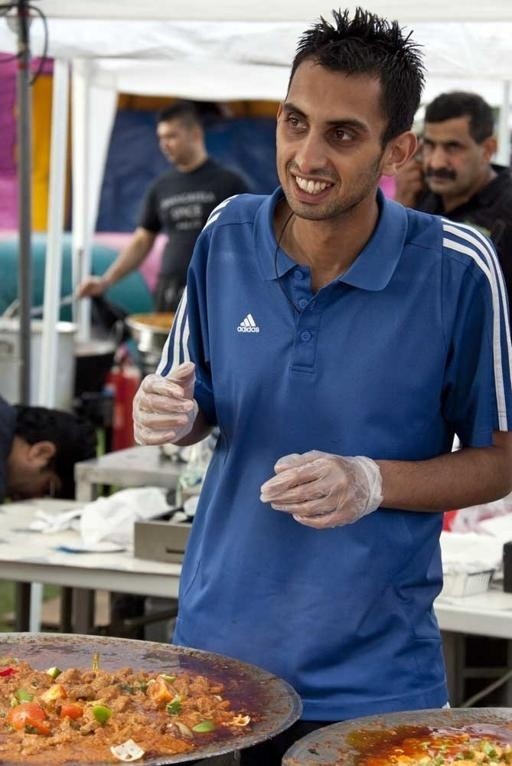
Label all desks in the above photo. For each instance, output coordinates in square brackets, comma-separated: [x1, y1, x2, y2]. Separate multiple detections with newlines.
[1, 496, 511, 707]
[73, 440, 197, 511]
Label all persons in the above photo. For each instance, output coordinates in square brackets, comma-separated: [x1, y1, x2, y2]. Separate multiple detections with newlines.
[73, 102, 254, 313]
[131, 6, 512, 766]
[393, 92, 512, 342]
[0, 395, 98, 506]
